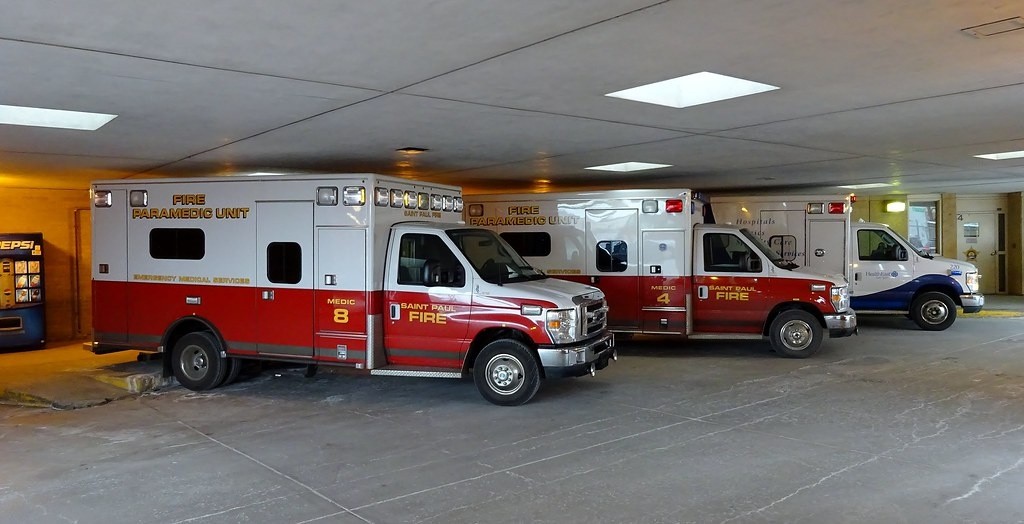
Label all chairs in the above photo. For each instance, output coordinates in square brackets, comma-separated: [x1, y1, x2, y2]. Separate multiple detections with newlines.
[424, 238, 461, 285]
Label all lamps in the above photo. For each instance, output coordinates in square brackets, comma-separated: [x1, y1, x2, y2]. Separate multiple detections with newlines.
[884, 200, 906, 213]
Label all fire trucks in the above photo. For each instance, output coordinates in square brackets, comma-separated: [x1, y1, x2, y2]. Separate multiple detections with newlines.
[84, 173, 618, 407]
[709, 194, 987, 332]
[458, 189, 858, 359]
